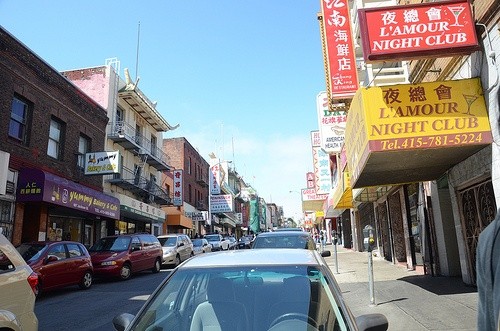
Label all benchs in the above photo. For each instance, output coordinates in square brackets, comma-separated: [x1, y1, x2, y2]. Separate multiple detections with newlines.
[190, 277, 324, 331]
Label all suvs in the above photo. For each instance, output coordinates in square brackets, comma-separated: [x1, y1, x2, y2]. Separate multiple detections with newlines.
[203, 232, 257, 250]
[88, 232, 163, 281]
[157, 234, 194, 268]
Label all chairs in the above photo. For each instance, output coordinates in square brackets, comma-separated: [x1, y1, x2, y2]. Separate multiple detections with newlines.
[267, 274, 326, 326]
[189, 278, 250, 331]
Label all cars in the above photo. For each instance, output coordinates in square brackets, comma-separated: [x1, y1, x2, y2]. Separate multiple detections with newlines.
[1, 241, 94, 299]
[0, 231, 38, 331]
[249, 228, 331, 272]
[190, 238, 213, 256]
[112, 248, 388, 331]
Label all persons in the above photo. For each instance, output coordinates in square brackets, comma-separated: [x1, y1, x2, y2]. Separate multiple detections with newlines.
[194, 233, 198, 239]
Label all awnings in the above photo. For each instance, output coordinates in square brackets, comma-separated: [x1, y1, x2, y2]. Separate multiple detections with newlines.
[324, 77, 493, 219]
[15, 169, 121, 220]
[162, 207, 192, 229]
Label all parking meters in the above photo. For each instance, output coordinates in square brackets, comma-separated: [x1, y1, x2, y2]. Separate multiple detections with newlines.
[319, 231, 325, 257]
[363, 225, 376, 307]
[331, 229, 341, 274]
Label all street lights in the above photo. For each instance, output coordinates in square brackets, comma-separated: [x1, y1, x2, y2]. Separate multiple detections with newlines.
[208, 160, 232, 234]
